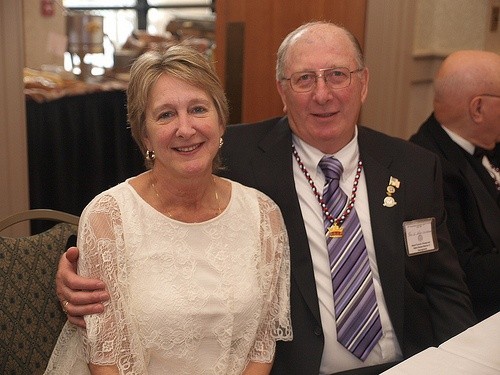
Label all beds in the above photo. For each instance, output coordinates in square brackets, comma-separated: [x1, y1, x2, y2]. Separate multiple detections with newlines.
[19, 29, 214, 148]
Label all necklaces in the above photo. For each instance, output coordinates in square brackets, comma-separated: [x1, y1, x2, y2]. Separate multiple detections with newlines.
[146, 172, 222, 221]
[291, 143, 363, 237]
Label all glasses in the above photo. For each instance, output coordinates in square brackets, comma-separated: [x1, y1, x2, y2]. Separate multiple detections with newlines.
[282, 66, 363, 93]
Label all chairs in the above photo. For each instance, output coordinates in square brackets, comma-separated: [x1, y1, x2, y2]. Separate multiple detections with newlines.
[0, 208, 88, 375]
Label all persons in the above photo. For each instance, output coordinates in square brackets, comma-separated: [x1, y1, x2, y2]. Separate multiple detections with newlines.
[56, 20, 479, 375]
[409, 51, 500, 323]
[42, 45, 293, 375]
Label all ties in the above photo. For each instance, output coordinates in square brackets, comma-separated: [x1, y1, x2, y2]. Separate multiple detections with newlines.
[318, 157, 383, 361]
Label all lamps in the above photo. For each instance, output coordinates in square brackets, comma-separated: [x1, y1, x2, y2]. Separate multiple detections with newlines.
[65, 13, 105, 74]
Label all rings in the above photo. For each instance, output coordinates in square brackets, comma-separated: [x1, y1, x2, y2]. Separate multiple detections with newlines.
[61, 301, 69, 314]
